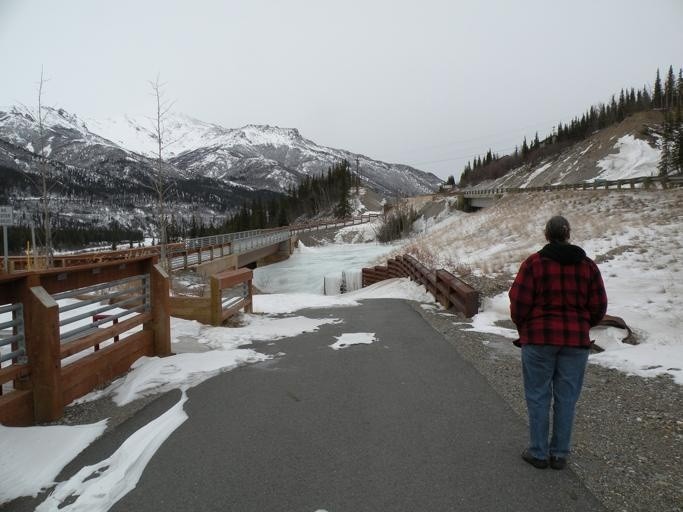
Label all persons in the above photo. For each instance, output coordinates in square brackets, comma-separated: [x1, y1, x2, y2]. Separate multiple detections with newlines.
[508, 216, 608, 470]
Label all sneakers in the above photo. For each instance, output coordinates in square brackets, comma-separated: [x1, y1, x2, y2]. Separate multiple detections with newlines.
[520, 448, 567, 470]
